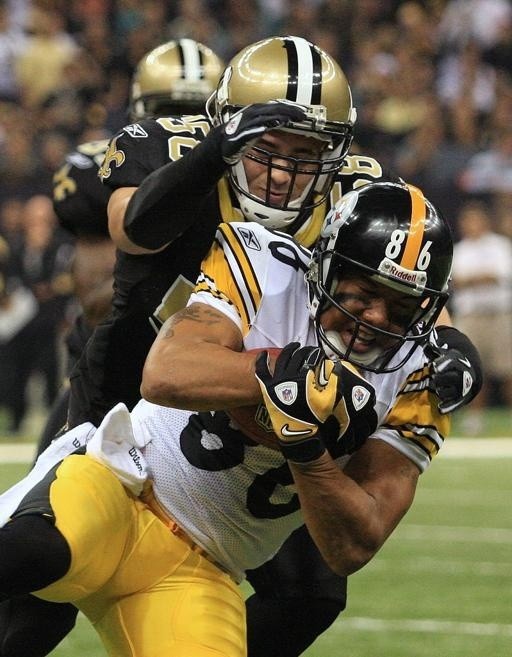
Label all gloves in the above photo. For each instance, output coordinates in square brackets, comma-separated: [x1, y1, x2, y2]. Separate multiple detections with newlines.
[212, 94, 308, 168]
[321, 356, 379, 460]
[428, 329, 487, 416]
[251, 339, 346, 463]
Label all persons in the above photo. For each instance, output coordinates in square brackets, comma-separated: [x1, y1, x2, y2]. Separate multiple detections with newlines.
[2, 35, 486, 656]
[0, 179, 453, 657]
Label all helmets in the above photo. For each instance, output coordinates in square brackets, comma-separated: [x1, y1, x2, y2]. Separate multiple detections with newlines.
[129, 36, 225, 118]
[216, 34, 358, 195]
[307, 181, 456, 317]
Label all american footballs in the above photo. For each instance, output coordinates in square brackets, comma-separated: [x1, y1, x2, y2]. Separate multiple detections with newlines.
[223, 347, 286, 451]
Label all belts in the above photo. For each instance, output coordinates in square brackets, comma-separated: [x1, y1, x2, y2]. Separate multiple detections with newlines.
[138, 478, 242, 584]
